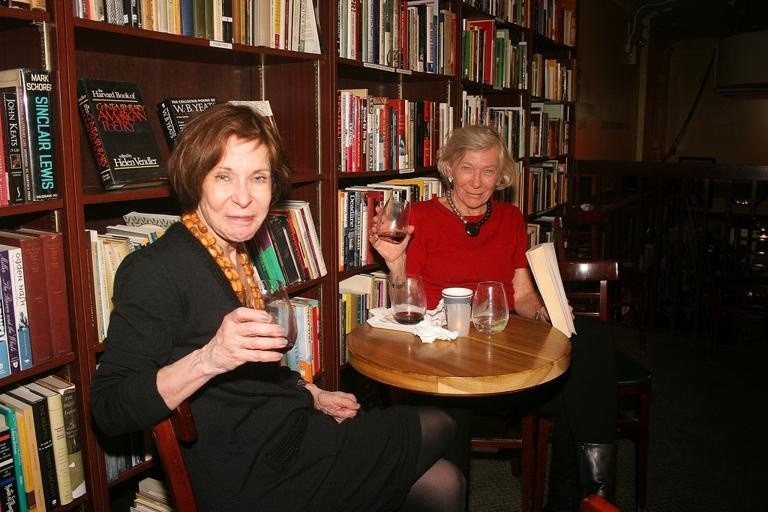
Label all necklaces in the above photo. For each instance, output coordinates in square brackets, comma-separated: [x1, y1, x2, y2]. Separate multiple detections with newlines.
[184, 213, 266, 312]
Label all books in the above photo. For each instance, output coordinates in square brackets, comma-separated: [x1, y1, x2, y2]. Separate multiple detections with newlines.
[77, 79, 279, 191]
[0, 67, 87, 512]
[104, 455, 173, 512]
[337, 1, 574, 366]
[2, 0, 323, 54]
[85, 210, 180, 342]
[244, 202, 328, 383]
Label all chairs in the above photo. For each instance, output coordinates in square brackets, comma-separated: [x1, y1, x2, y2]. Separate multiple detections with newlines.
[460, 404, 537, 512]
[150, 400, 199, 511]
[503, 253, 655, 512]
[552, 208, 650, 361]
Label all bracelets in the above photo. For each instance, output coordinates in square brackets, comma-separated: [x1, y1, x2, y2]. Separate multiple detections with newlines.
[298, 380, 309, 386]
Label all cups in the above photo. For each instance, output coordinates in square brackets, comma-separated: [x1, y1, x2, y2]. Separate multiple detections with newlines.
[441, 287, 473, 336]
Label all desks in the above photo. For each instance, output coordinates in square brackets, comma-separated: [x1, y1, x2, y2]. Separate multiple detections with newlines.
[346, 310, 574, 512]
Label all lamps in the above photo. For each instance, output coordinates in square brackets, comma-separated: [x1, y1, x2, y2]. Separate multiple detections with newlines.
[620, 1, 676, 66]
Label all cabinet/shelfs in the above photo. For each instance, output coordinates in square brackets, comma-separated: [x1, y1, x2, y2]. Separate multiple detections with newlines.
[0, 1, 100, 511]
[650, 175, 768, 344]
[331, 0, 461, 407]
[64, 0, 335, 512]
[461, 0, 577, 260]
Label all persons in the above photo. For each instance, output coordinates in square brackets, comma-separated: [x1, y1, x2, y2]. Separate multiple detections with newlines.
[88, 105, 467, 512]
[366, 123, 619, 512]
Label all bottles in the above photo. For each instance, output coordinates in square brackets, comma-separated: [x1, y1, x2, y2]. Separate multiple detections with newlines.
[375, 195, 411, 244]
[241, 278, 296, 354]
[385, 274, 428, 325]
[471, 281, 509, 335]
